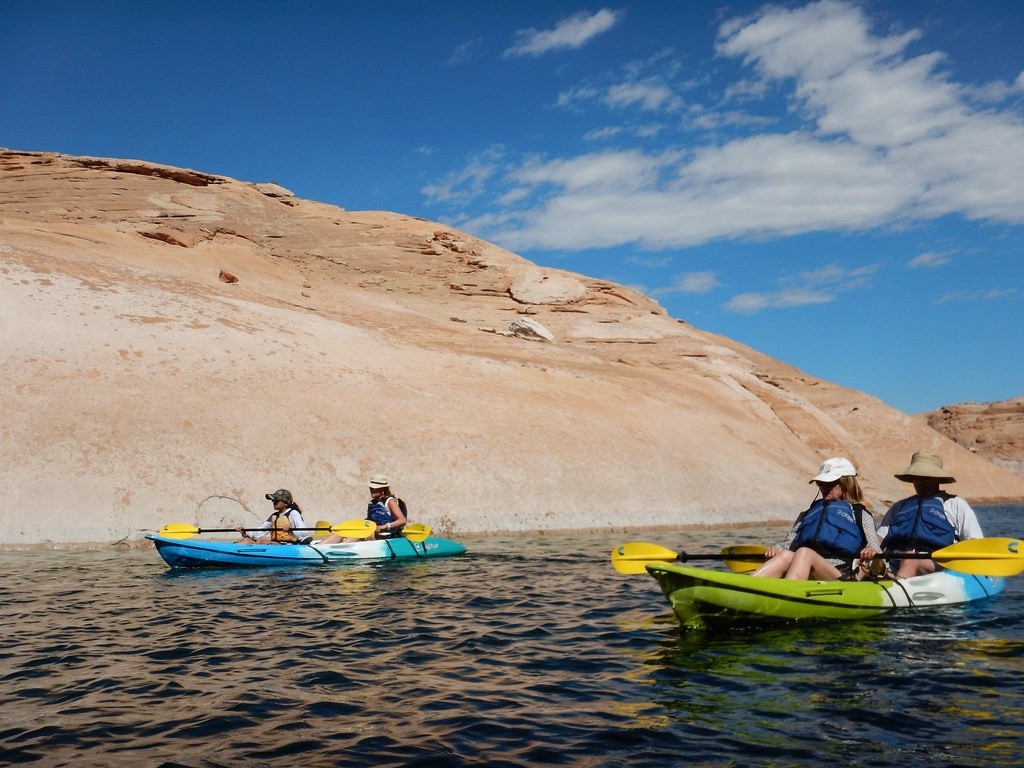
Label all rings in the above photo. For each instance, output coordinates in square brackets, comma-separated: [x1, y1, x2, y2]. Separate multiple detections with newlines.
[768, 552, 771, 554]
[765, 553, 768, 556]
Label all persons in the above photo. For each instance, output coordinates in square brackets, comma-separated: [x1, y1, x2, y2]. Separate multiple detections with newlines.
[317, 474, 407, 544]
[751, 457, 888, 581]
[875, 451, 984, 580]
[234, 489, 313, 545]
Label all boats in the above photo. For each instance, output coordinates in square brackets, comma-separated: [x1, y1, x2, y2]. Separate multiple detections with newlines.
[148, 531, 468, 570]
[645, 560, 1007, 636]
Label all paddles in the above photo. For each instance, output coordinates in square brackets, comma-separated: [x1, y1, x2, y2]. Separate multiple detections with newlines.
[157, 519, 377, 540]
[719, 543, 777, 575]
[314, 520, 432, 543]
[608, 539, 1024, 576]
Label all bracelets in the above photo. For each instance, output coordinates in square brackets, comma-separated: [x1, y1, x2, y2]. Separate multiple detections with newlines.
[387, 523, 390, 530]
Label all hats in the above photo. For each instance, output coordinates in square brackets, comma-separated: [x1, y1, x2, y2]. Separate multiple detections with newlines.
[895, 450, 957, 484]
[368, 474, 391, 489]
[808, 456, 857, 484]
[265, 489, 292, 504]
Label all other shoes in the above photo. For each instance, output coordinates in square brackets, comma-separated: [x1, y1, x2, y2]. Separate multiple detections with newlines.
[300, 536, 313, 545]
